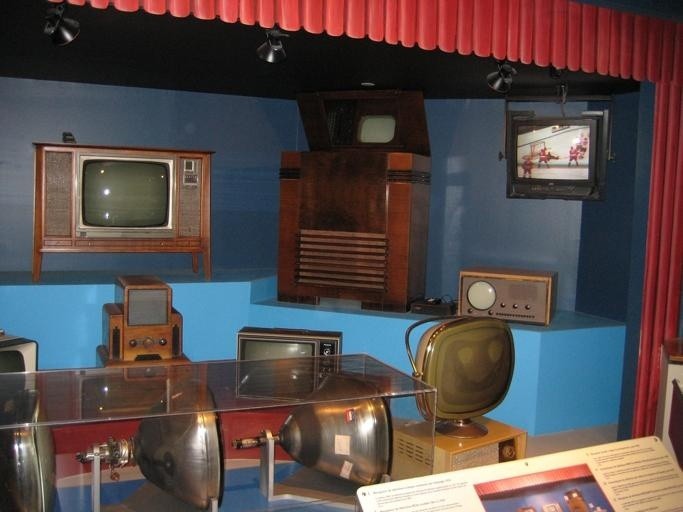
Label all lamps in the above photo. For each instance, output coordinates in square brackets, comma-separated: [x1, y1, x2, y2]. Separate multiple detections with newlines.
[42, 1, 82, 47]
[255, 27, 294, 64]
[482, 59, 520, 95]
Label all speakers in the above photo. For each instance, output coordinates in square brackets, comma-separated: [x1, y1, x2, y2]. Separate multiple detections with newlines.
[391, 415, 528, 481]
[177, 157, 201, 238]
[96, 275, 194, 414]
[44, 151, 72, 236]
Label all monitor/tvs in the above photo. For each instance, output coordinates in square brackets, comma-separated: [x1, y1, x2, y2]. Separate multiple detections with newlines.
[505, 109, 612, 201]
[235, 326, 343, 403]
[0, 330, 39, 393]
[404, 315, 515, 439]
[76, 153, 177, 240]
[1, 390, 55, 512]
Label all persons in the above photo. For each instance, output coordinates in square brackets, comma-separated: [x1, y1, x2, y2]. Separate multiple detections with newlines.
[521, 158, 534, 178]
[567, 131, 588, 168]
[537, 146, 551, 169]
